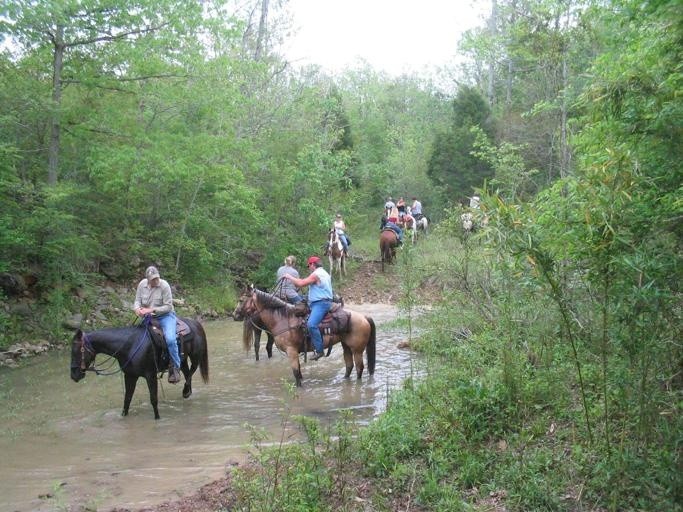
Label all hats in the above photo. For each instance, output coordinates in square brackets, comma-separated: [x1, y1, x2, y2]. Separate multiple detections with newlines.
[307, 256, 321, 265]
[145, 266, 160, 281]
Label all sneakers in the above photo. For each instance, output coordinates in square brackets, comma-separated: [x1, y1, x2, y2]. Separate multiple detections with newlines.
[168, 370, 181, 383]
[310, 351, 324, 361]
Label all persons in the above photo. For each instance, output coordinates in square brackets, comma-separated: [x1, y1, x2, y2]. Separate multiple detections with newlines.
[324, 214, 349, 258]
[470, 192, 481, 209]
[380, 197, 422, 245]
[277, 256, 302, 302]
[133, 266, 181, 383]
[282, 257, 333, 360]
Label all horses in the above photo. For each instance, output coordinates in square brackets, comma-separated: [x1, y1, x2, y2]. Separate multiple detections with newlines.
[70, 317, 209, 420]
[243, 294, 345, 361]
[232, 283, 376, 388]
[327, 227, 348, 281]
[402, 214, 417, 245]
[380, 214, 404, 273]
[406, 205, 428, 234]
